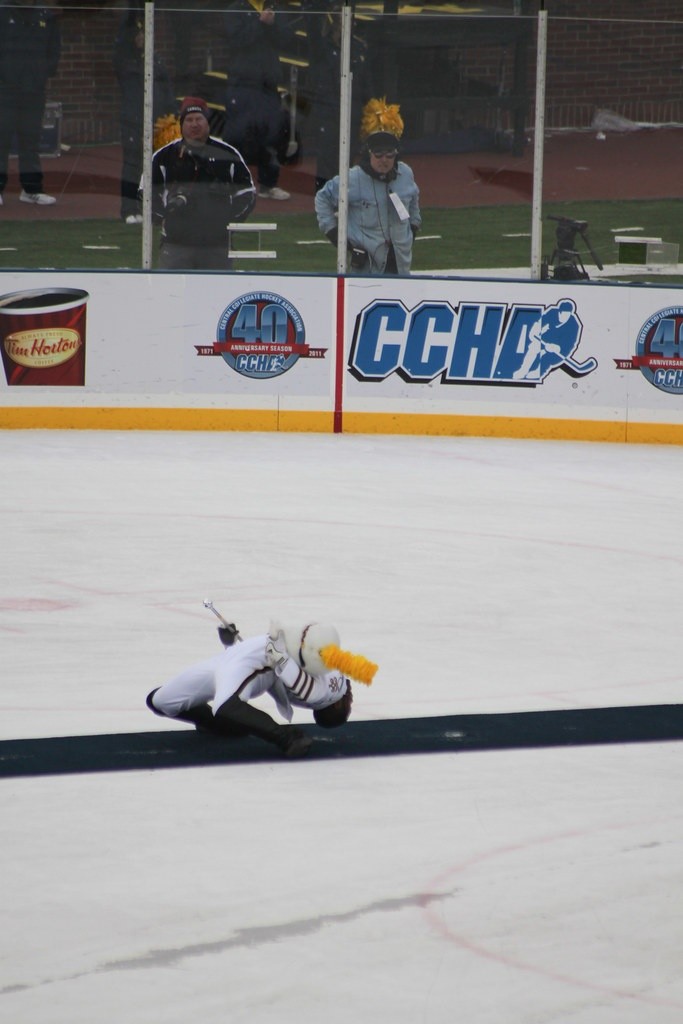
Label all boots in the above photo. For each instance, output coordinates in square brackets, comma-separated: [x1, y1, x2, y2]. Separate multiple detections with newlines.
[211, 694, 313, 756]
[145, 684, 249, 741]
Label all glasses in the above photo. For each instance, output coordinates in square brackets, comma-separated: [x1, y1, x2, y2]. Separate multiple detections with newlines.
[370, 150, 399, 159]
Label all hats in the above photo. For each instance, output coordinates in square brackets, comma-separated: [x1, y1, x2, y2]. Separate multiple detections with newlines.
[361, 96, 404, 147]
[179, 95, 210, 127]
[284, 623, 378, 685]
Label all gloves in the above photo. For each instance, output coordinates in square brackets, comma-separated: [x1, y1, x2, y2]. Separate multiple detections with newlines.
[217, 624, 240, 650]
[265, 625, 290, 666]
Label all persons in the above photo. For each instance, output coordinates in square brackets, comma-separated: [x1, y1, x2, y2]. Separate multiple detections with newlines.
[219, 0, 388, 201]
[0, 0, 57, 205]
[314, 96, 422, 279]
[134, 95, 256, 269]
[113, 1, 179, 226]
[146, 619, 354, 759]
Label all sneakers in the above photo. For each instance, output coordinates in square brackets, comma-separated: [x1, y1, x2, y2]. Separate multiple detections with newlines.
[19, 187, 56, 204]
[125, 212, 144, 224]
[258, 184, 290, 200]
[0, 191, 6, 206]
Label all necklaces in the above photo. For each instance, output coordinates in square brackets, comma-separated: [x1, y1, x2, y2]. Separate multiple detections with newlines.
[371, 175, 391, 248]
